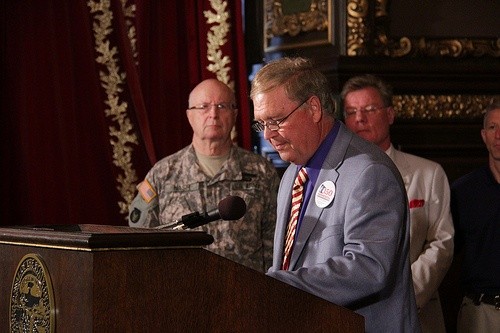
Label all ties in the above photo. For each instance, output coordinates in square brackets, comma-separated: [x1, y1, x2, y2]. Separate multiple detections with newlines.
[279, 165, 309, 270]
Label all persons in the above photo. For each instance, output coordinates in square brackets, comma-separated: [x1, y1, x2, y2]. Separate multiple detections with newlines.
[249, 56, 419, 333]
[128, 78, 280, 274]
[335, 74, 455, 333]
[436, 107, 500, 303]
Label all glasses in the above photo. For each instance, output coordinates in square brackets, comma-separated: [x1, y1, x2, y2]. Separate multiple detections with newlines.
[344, 105, 386, 119]
[188, 103, 235, 111]
[252, 98, 310, 131]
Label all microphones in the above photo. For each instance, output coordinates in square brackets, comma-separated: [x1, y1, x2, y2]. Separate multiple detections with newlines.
[174, 196, 246, 230]
